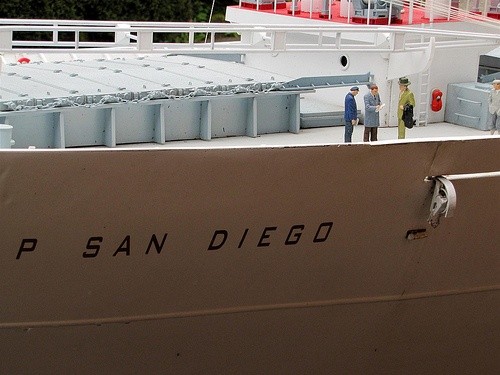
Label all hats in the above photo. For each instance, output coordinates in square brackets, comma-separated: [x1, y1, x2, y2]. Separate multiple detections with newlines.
[350, 87, 359, 91]
[397, 77, 411, 85]
[492, 80, 500, 85]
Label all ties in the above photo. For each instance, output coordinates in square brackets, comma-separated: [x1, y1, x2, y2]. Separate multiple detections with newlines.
[373, 95, 375, 99]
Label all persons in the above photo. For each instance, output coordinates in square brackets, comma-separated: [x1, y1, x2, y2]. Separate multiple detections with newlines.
[398, 77, 415, 139]
[345, 87, 359, 143]
[488, 80, 500, 135]
[363, 84, 383, 142]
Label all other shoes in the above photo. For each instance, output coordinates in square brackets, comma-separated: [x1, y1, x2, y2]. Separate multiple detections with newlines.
[489, 130, 495, 135]
